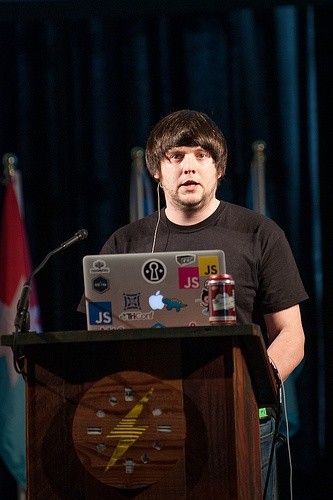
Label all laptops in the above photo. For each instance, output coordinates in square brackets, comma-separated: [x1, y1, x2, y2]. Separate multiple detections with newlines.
[82, 250, 226, 332]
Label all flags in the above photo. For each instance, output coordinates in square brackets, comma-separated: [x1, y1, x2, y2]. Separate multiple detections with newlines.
[0, 157, 42, 387]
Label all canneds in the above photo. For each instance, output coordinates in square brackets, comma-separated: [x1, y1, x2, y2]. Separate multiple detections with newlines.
[207, 274, 236, 326]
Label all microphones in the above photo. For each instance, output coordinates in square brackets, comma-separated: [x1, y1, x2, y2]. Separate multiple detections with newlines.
[61, 230, 89, 249]
[160, 185, 168, 189]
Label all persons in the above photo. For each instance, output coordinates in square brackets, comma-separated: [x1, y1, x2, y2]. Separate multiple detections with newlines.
[76, 111, 304, 500]
